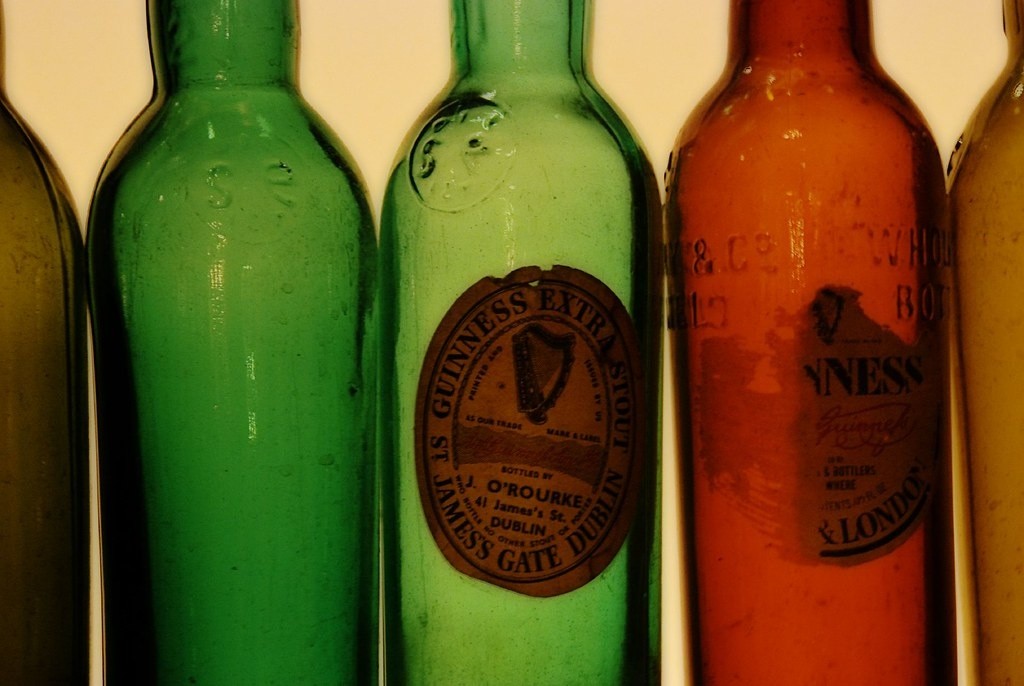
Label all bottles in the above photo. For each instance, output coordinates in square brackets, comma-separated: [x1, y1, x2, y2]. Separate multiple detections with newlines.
[662, 0, 959, 686]
[947, 0, 1024, 686]
[0, 100, 91, 686]
[379, 0, 661, 686]
[85, 0, 377, 686]
